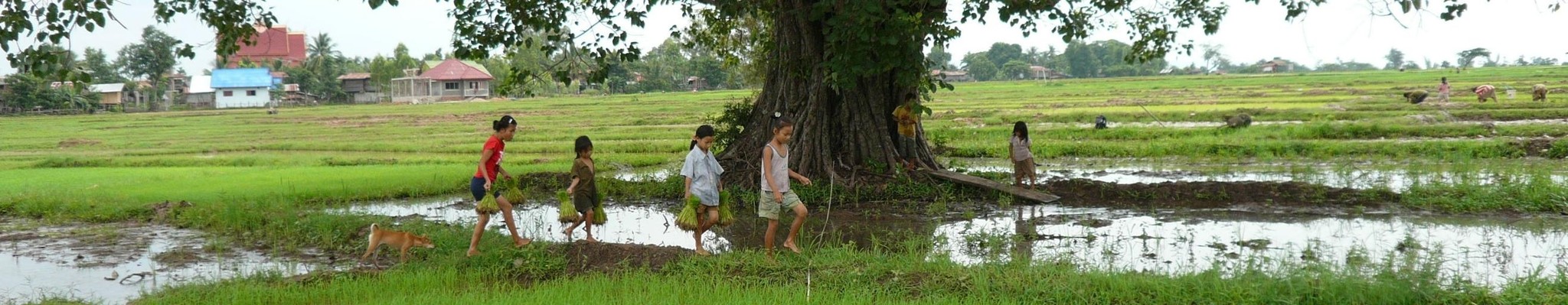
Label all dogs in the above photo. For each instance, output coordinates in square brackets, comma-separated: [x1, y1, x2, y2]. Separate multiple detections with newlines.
[357, 223, 434, 271]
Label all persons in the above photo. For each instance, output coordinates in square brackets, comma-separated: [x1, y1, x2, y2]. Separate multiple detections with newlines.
[1404, 90, 1428, 104]
[679, 126, 725, 257]
[467, 116, 535, 256]
[1532, 85, 1547, 102]
[1438, 77, 1450, 103]
[758, 111, 813, 258]
[1473, 85, 1499, 103]
[892, 93, 918, 168]
[1009, 121, 1036, 190]
[564, 136, 599, 242]
